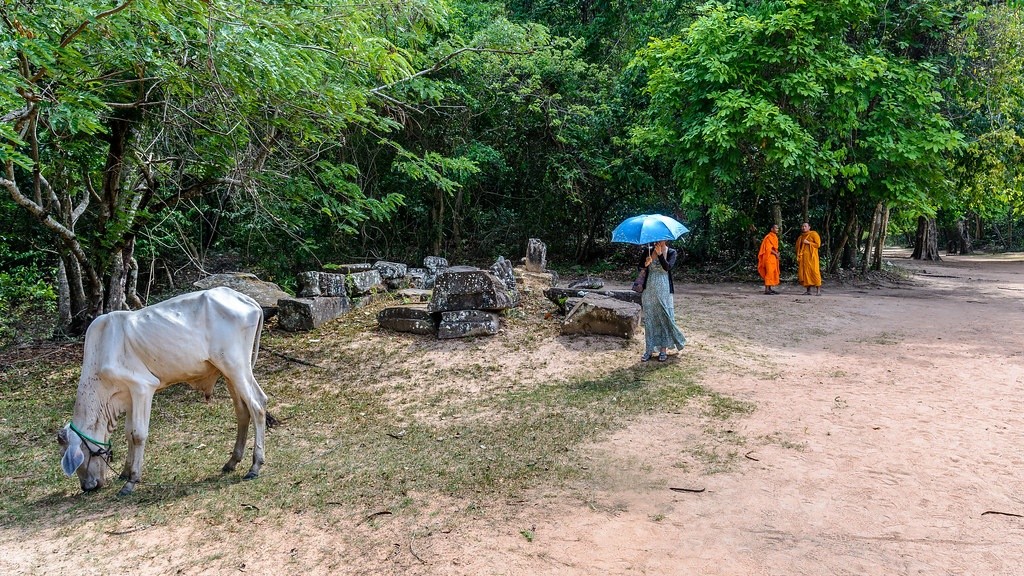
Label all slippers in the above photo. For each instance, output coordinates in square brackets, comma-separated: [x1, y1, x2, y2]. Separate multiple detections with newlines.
[763, 291, 775, 295]
[641, 353, 652, 361]
[659, 352, 668, 362]
[771, 289, 779, 294]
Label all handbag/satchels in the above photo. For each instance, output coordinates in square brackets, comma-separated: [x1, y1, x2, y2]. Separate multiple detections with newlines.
[631, 267, 648, 294]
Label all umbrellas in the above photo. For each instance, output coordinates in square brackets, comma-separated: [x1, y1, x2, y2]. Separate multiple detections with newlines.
[610, 213, 690, 257]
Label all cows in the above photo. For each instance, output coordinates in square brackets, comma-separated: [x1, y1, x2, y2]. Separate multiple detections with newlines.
[57, 286, 268, 495]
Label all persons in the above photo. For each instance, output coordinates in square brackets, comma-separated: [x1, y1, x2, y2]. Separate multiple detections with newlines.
[758, 224, 781, 295]
[795, 222, 822, 296]
[638, 241, 686, 362]
